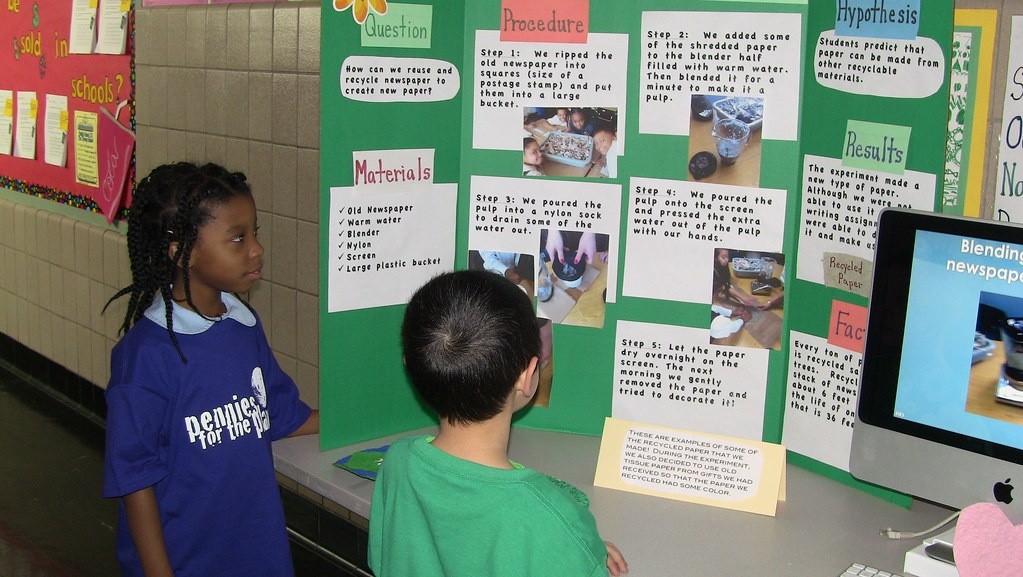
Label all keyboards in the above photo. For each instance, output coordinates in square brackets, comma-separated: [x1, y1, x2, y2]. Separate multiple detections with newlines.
[838, 563, 903, 577]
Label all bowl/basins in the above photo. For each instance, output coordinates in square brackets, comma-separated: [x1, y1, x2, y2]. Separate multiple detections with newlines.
[712, 96, 763, 131]
[538, 131, 594, 167]
[732, 257, 761, 277]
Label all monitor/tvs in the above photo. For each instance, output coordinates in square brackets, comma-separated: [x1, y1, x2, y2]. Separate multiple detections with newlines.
[848, 205, 1023, 576]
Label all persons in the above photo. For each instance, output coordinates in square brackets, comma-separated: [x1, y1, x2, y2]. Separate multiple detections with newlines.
[368, 269, 629, 577]
[710, 250, 785, 344]
[523, 107, 617, 178]
[479, 250, 521, 283]
[545, 229, 597, 265]
[103, 163, 320, 577]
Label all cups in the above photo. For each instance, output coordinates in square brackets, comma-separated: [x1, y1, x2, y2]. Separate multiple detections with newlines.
[712, 118, 751, 164]
[760, 257, 777, 279]
[551, 250, 586, 287]
[999, 316, 1023, 386]
[538, 255, 553, 302]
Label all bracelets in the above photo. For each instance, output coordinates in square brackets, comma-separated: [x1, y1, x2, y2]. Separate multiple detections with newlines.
[768, 300, 771, 304]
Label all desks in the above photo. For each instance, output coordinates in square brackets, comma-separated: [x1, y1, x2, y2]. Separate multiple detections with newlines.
[266, 425, 962, 577]
